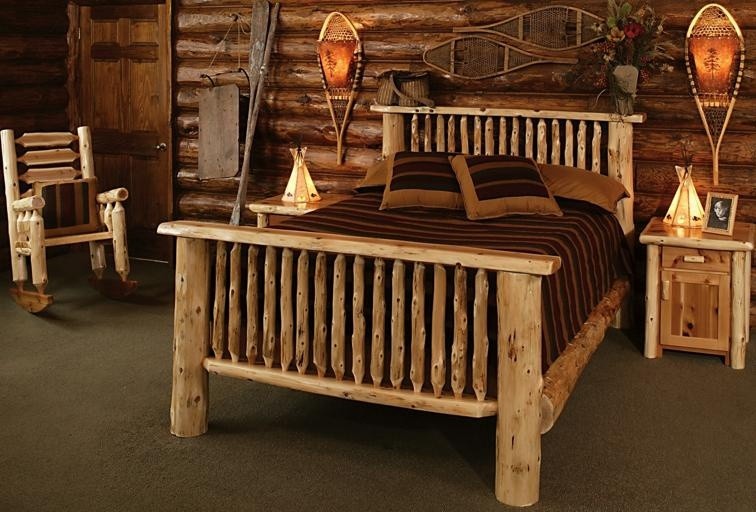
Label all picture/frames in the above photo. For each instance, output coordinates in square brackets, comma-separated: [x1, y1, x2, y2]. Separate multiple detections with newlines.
[702, 191, 740, 237]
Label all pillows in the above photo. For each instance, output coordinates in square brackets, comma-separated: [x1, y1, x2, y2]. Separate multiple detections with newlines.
[355, 150, 630, 222]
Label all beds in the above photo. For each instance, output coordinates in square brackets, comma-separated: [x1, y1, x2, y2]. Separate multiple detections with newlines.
[157, 105, 650, 506]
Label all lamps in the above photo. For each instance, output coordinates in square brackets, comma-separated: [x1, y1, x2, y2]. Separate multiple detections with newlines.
[314, 12, 363, 164]
[685, 5, 745, 188]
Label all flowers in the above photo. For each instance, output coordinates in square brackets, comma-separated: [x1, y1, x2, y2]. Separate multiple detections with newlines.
[285, 130, 307, 164]
[552, 1, 681, 131]
[665, 137, 701, 168]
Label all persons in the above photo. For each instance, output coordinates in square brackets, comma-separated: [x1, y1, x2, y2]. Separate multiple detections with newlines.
[707, 200, 729, 231]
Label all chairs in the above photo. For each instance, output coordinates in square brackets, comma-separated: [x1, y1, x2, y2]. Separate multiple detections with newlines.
[0, 125, 145, 314]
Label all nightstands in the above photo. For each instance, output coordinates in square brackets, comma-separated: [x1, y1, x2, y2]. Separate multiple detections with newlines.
[639, 216, 755, 370]
[247, 192, 352, 228]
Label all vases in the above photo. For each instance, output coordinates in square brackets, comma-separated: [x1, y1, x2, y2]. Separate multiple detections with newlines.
[281, 147, 324, 204]
[665, 164, 705, 229]
[613, 63, 640, 116]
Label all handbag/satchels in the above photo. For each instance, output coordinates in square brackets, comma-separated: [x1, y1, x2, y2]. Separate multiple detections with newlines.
[377, 70, 430, 107]
